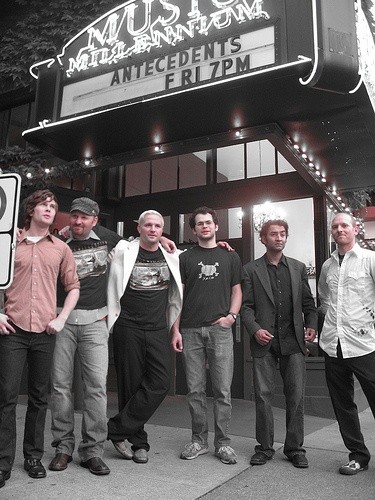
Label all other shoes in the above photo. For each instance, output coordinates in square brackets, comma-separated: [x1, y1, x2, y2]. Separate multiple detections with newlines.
[133, 449, 148, 463]
[107, 436, 133, 459]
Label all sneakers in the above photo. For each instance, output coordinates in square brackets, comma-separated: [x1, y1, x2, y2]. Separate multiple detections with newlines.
[214, 447, 237, 464]
[179, 441, 208, 460]
[338, 459, 369, 475]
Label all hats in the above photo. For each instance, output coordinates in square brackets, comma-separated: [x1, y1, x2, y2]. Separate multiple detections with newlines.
[70, 197, 100, 217]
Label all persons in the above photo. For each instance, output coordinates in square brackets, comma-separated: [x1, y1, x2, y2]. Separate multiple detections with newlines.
[16, 197, 176, 475]
[107, 210, 235, 463]
[318, 212, 375, 475]
[242, 219, 318, 469]
[0, 189, 80, 489]
[170, 206, 244, 465]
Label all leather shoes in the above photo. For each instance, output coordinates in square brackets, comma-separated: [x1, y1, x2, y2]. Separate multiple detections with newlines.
[250, 451, 273, 465]
[80, 457, 110, 474]
[48, 453, 71, 471]
[24, 459, 46, 478]
[0, 469, 11, 488]
[288, 454, 309, 467]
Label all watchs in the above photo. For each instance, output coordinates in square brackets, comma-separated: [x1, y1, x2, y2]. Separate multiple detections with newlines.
[229, 313, 237, 319]
